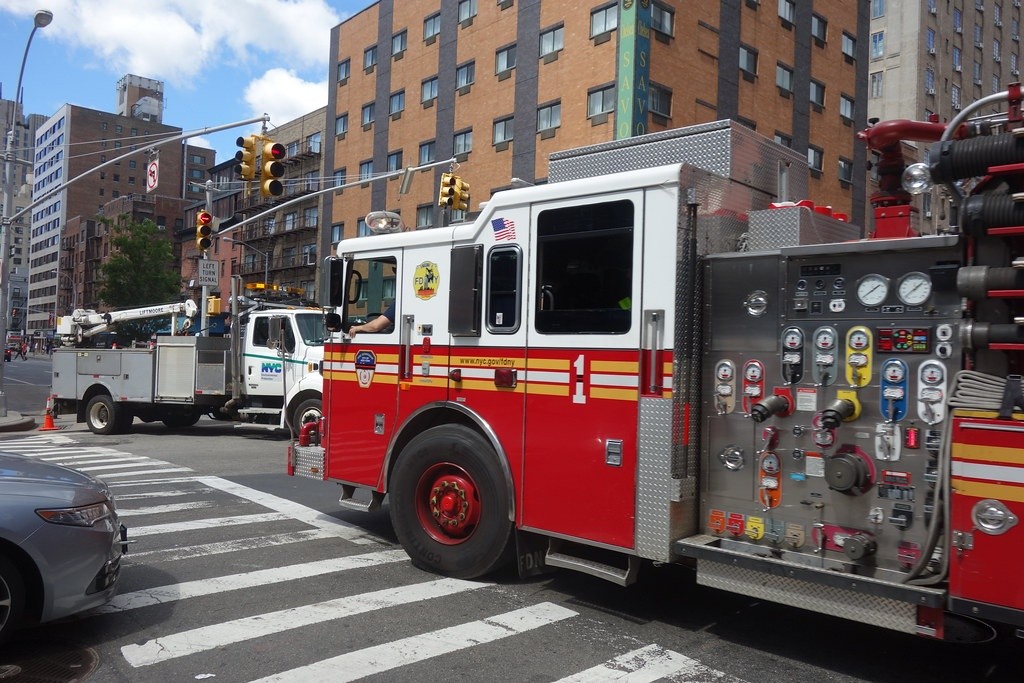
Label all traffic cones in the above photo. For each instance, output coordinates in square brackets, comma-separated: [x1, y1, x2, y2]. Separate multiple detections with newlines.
[38, 397, 60, 430]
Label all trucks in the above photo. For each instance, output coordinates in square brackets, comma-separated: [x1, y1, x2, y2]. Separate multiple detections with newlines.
[51, 282, 348, 446]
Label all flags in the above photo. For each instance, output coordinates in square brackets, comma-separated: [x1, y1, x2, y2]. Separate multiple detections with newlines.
[491, 218, 517, 241]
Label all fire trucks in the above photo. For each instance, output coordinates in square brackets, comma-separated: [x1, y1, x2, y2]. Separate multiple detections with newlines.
[302, 79, 1024, 683]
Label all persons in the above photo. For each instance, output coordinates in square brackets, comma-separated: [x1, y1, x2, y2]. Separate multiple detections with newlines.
[349, 267, 396, 338]
[11, 341, 60, 361]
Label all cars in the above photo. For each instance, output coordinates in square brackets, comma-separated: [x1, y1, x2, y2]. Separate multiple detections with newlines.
[1, 446, 137, 652]
[5, 343, 12, 362]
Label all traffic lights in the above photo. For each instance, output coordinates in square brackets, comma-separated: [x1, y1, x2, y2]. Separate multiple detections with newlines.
[12, 308, 17, 318]
[260, 140, 287, 199]
[438, 172, 456, 207]
[452, 178, 469, 212]
[195, 209, 213, 251]
[234, 136, 256, 180]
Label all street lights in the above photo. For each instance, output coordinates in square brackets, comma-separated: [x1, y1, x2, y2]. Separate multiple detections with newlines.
[223, 236, 270, 299]
[0, 8, 53, 417]
[50, 270, 76, 310]
